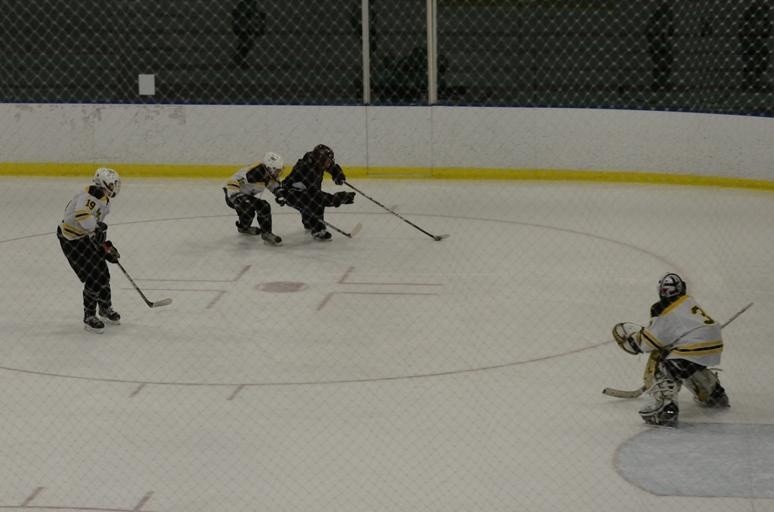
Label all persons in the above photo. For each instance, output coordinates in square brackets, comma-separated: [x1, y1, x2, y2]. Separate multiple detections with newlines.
[612, 273, 729, 426]
[282, 144, 356, 239]
[222, 152, 285, 246]
[57, 168, 120, 328]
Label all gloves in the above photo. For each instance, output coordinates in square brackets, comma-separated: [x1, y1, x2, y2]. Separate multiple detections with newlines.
[290, 182, 307, 192]
[331, 163, 345, 186]
[334, 190, 356, 207]
[97, 239, 120, 265]
[275, 191, 287, 206]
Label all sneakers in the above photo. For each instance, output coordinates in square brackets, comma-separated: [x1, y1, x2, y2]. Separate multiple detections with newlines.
[97, 303, 120, 321]
[83, 307, 105, 329]
[259, 230, 281, 243]
[236, 221, 262, 236]
[642, 404, 679, 425]
[694, 383, 727, 408]
[301, 219, 316, 231]
[312, 228, 331, 239]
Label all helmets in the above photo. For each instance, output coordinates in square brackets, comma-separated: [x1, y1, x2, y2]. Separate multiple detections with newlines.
[312, 144, 335, 169]
[264, 150, 284, 172]
[657, 270, 687, 301]
[92, 167, 122, 198]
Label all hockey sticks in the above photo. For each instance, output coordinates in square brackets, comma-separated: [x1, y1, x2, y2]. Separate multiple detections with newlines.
[342, 178, 451, 242]
[601, 303, 754, 399]
[115, 259, 172, 309]
[283, 202, 364, 239]
